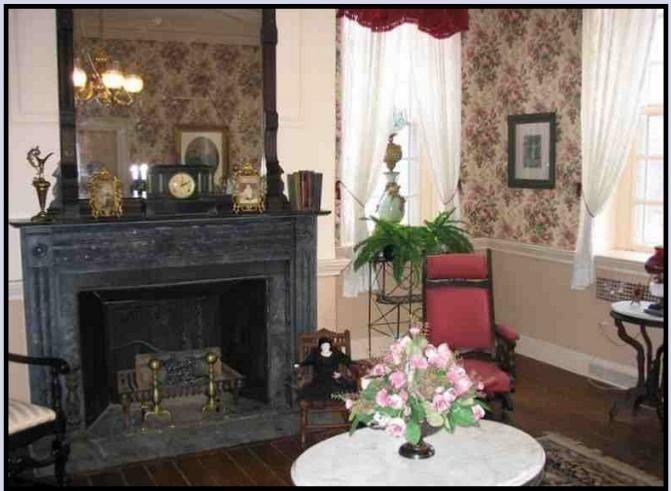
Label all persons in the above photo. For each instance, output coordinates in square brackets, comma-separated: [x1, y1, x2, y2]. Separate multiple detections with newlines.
[291, 337, 362, 408]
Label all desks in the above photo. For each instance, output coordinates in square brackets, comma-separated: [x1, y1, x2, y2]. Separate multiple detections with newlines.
[291, 417, 548, 487]
[610, 300, 667, 445]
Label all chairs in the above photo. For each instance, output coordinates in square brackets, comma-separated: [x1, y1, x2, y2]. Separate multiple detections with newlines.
[420, 248, 520, 421]
[296, 328, 360, 450]
[7, 354, 74, 491]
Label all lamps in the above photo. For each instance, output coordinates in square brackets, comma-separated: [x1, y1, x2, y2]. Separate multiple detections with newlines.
[375, 133, 407, 224]
[643, 246, 664, 317]
[68, 9, 145, 109]
[127, 158, 149, 198]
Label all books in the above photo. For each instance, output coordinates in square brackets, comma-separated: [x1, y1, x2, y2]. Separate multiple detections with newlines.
[286, 168, 323, 210]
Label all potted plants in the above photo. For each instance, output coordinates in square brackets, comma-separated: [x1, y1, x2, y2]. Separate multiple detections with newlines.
[352, 205, 475, 289]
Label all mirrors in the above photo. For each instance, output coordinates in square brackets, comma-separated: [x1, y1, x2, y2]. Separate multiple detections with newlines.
[48, 5, 291, 223]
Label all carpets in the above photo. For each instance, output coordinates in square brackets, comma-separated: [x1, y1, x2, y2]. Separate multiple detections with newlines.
[523, 431, 665, 487]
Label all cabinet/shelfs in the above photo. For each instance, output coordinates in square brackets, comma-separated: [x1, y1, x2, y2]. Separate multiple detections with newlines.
[365, 256, 424, 358]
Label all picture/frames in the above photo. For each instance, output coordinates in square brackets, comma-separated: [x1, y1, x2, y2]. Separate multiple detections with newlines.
[507, 113, 556, 189]
[228, 161, 265, 213]
[86, 169, 125, 220]
[172, 124, 230, 193]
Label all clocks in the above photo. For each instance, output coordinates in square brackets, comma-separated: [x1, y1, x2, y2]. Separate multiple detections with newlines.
[144, 164, 231, 219]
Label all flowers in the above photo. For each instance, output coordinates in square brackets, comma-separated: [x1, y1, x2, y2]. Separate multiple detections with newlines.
[340, 332, 491, 446]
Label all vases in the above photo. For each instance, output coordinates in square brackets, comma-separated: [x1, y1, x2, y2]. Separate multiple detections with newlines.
[398, 416, 444, 459]
